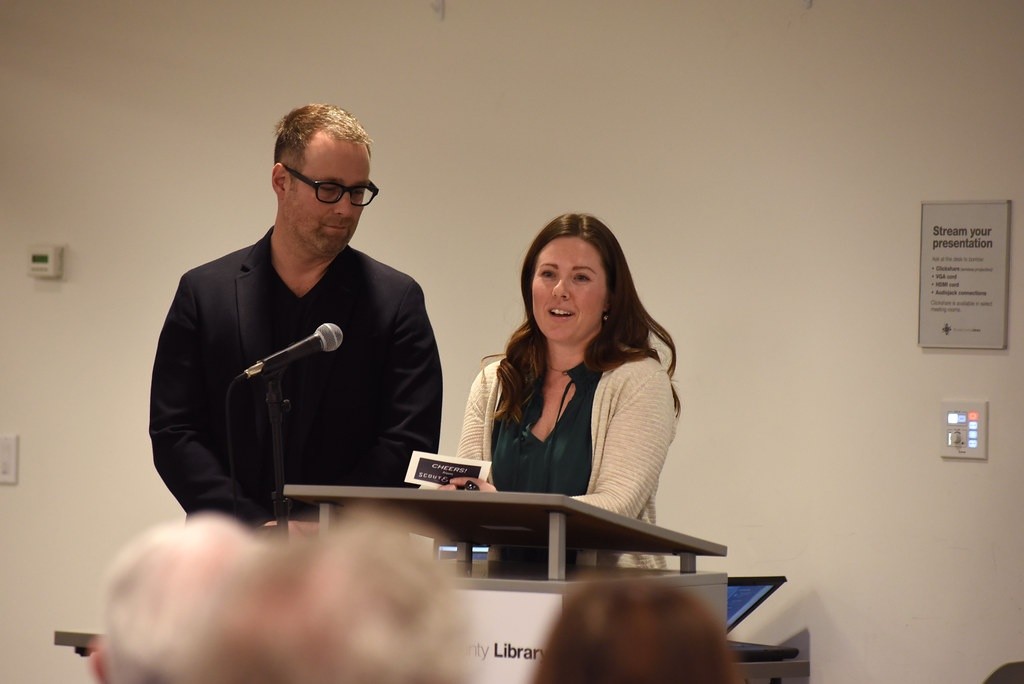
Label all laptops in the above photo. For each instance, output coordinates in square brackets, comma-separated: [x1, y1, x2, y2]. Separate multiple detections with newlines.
[727, 576, 800, 662]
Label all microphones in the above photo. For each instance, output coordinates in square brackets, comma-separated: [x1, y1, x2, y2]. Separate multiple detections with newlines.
[243, 323, 344, 380]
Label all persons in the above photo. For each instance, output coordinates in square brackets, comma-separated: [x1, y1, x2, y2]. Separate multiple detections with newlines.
[88, 510, 744, 684]
[149, 104, 444, 544]
[437, 213, 681, 572]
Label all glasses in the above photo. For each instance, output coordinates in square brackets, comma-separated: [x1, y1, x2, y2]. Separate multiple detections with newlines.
[283, 166, 379, 206]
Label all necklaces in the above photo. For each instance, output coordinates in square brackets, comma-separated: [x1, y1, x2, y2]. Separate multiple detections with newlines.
[545, 358, 569, 376]
